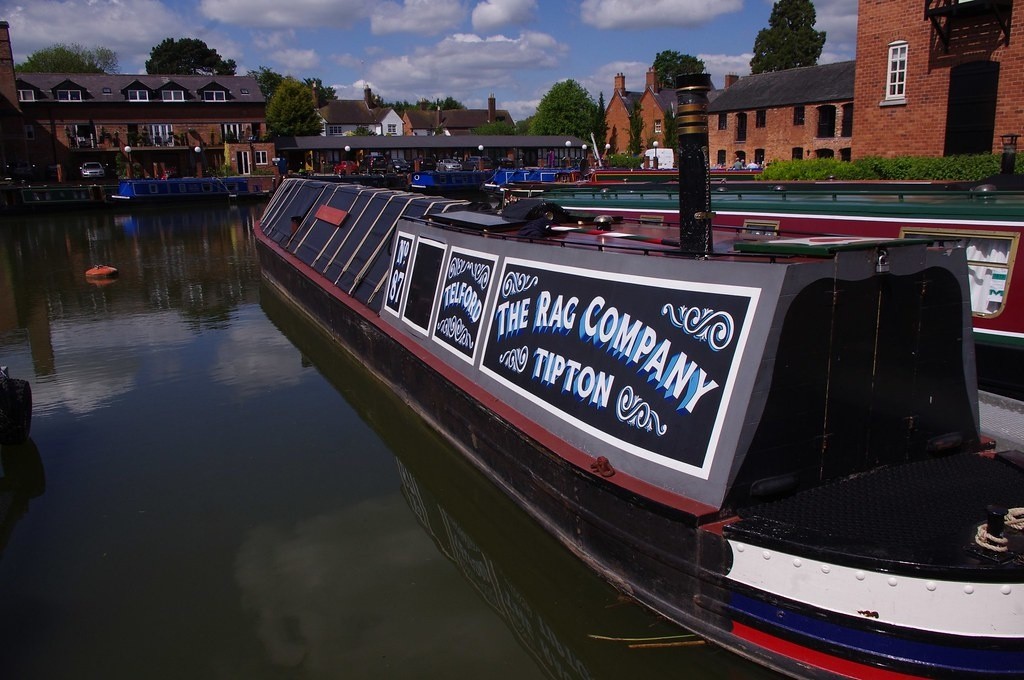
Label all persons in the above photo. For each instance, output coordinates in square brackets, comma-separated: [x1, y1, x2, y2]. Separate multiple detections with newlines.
[432, 152, 437, 170]
[732, 158, 761, 169]
[549, 148, 555, 168]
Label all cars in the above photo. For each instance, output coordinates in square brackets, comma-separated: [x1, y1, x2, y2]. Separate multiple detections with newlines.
[388, 158, 411, 174]
[413, 157, 436, 171]
[333, 160, 359, 175]
[497, 157, 515, 170]
[437, 159, 463, 172]
[79, 162, 106, 180]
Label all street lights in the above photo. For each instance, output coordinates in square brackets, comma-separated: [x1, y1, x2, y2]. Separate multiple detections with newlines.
[605, 143, 611, 168]
[345, 145, 351, 175]
[478, 145, 484, 172]
[581, 144, 587, 159]
[125, 145, 132, 179]
[652, 141, 659, 171]
[248, 135, 257, 174]
[566, 141, 572, 160]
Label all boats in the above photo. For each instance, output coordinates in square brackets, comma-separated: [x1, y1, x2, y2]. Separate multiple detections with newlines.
[253, 75, 1023, 679]
[111, 177, 251, 203]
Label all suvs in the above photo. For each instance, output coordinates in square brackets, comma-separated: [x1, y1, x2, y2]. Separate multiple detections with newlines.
[360, 154, 388, 175]
[463, 156, 494, 172]
